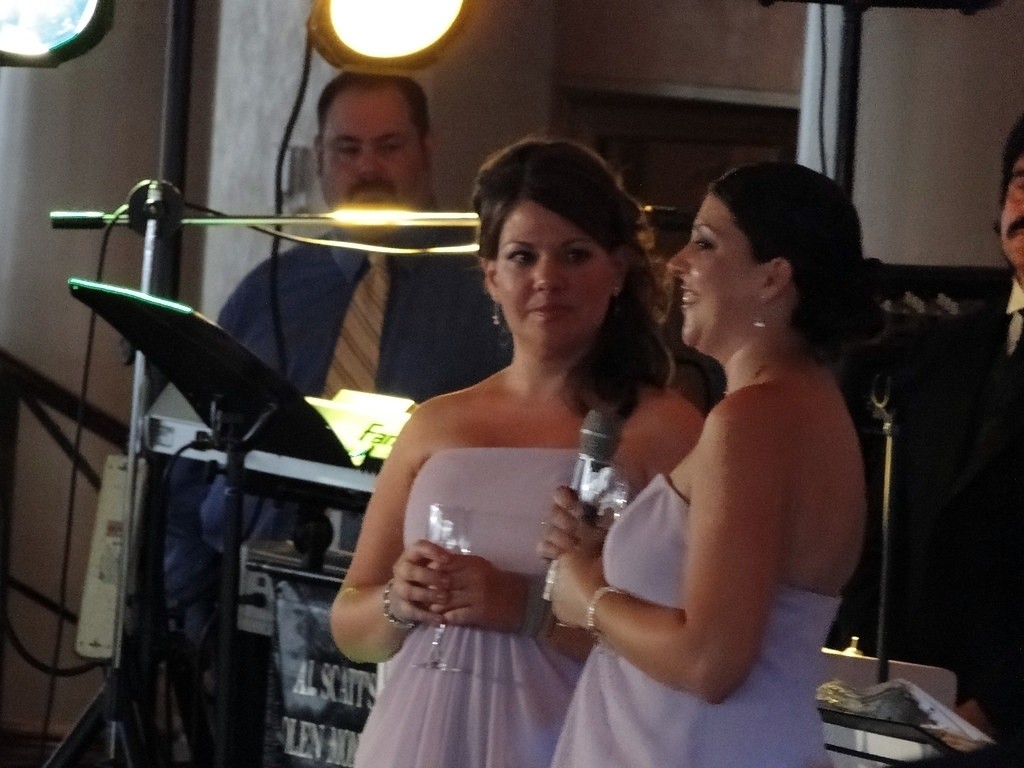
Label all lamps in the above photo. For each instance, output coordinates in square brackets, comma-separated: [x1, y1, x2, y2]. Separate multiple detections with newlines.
[0, 0, 117, 69]
[304, 0, 473, 71]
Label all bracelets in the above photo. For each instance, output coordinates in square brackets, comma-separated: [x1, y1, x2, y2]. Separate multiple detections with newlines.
[383, 581, 416, 629]
[588, 586, 622, 628]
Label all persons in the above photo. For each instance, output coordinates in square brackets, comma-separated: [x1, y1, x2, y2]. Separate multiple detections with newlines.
[836, 116, 1024, 768]
[161, 72, 515, 768]
[537, 163, 889, 768]
[329, 137, 703, 768]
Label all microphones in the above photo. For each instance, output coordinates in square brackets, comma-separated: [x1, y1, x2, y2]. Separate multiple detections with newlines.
[546, 406, 625, 600]
